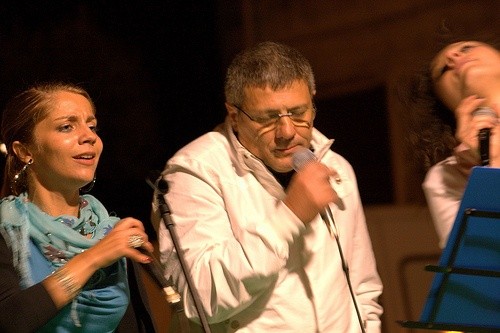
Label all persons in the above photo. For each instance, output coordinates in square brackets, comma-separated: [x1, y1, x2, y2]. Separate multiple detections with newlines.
[150, 42, 385, 333]
[407, 24, 500, 252]
[1, 84, 155, 332]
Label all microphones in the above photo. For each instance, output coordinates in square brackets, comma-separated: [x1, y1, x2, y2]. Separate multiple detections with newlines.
[472, 107, 494, 165]
[290, 147, 338, 239]
[136, 246, 181, 305]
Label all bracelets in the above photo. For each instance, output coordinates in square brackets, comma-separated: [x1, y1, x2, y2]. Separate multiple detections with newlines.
[51, 267, 81, 303]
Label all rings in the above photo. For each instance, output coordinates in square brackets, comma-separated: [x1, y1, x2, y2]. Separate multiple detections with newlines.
[127, 234, 145, 249]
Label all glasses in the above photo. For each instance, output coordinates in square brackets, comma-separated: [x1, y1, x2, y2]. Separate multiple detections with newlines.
[233, 104, 317, 123]
